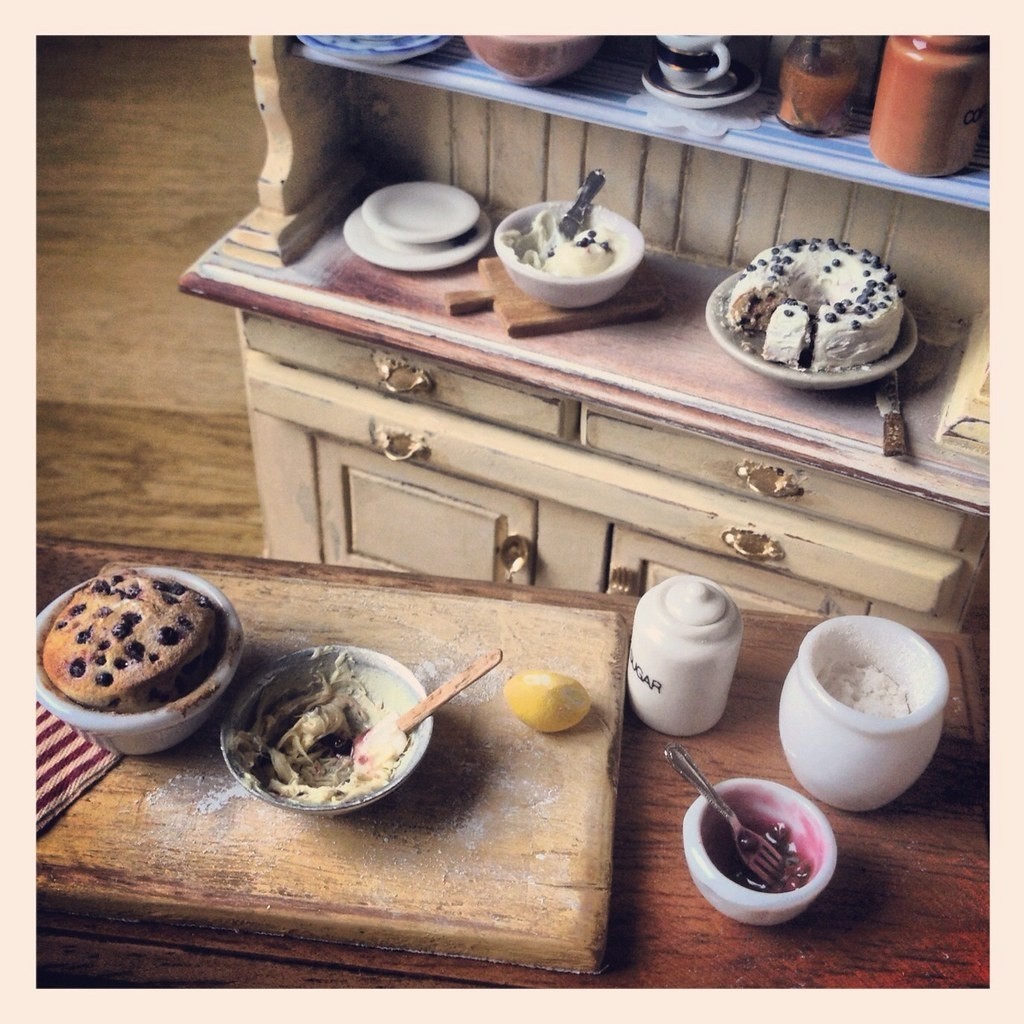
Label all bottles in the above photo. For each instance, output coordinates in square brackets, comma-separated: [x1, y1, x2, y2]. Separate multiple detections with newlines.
[626, 574, 744, 737]
[779, 615, 950, 814]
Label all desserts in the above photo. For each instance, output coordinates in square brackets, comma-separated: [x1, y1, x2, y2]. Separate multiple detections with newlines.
[499, 209, 631, 277]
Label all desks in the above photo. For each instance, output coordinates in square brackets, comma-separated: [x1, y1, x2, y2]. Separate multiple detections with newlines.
[37, 536, 991, 989]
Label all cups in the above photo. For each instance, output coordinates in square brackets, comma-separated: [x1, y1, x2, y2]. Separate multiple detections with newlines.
[655, 35, 734, 93]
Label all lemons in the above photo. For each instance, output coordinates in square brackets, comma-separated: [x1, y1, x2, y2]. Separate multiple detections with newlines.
[504, 670, 591, 732]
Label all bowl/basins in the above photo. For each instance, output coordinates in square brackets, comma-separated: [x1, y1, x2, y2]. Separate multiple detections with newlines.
[219, 645, 434, 814]
[493, 200, 646, 309]
[35, 566, 245, 756]
[680, 776, 837, 924]
[704, 256, 919, 393]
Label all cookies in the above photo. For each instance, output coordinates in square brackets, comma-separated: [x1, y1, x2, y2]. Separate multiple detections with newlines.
[41, 567, 219, 710]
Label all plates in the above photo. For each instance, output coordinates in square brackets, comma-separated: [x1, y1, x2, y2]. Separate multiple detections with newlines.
[361, 179, 482, 247]
[343, 202, 491, 273]
[296, 35, 457, 66]
[641, 63, 763, 109]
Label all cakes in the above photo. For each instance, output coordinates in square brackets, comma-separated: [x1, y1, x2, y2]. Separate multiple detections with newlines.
[727, 237, 908, 370]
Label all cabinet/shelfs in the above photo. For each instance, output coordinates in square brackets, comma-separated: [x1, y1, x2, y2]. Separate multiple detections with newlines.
[179, 34, 991, 633]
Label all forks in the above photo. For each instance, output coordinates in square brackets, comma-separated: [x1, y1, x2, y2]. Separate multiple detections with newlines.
[665, 741, 783, 888]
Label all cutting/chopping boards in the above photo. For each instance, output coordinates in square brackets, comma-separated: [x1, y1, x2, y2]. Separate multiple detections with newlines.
[38, 569, 630, 975]
